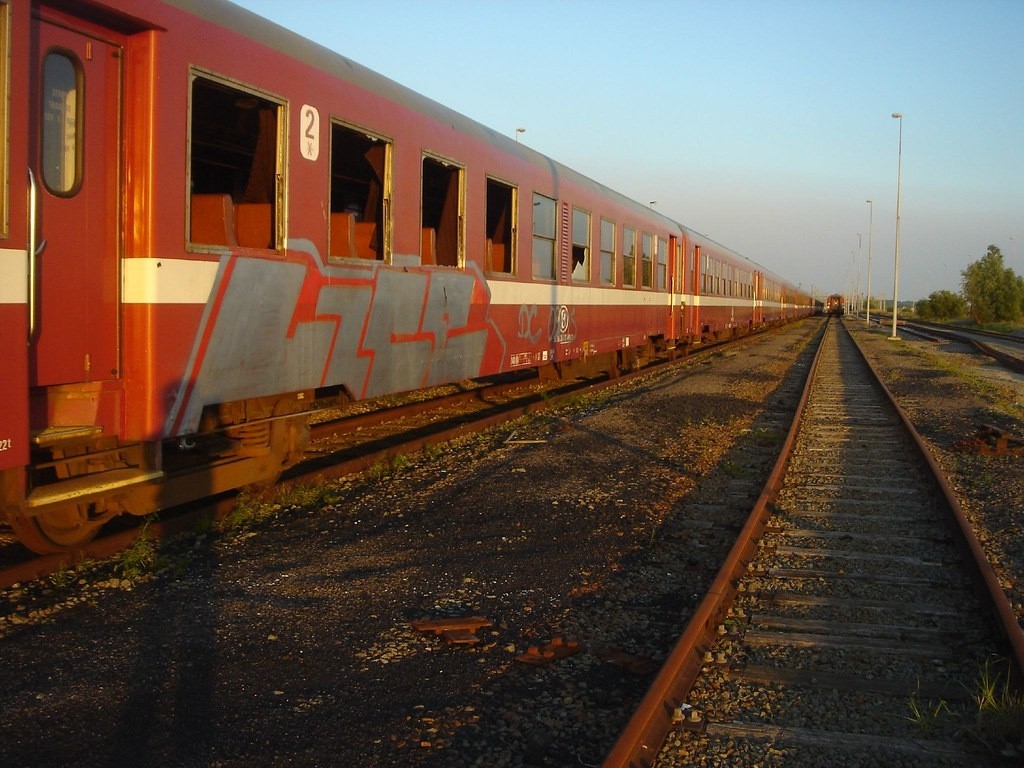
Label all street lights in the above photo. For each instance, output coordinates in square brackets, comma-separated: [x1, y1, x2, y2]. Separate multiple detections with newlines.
[516, 127, 525, 143]
[857, 232, 862, 306]
[887, 112, 903, 342]
[866, 200, 872, 326]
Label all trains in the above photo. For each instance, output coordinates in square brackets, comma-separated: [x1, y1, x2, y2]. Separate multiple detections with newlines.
[827, 294, 845, 316]
[0, 0, 824, 555]
